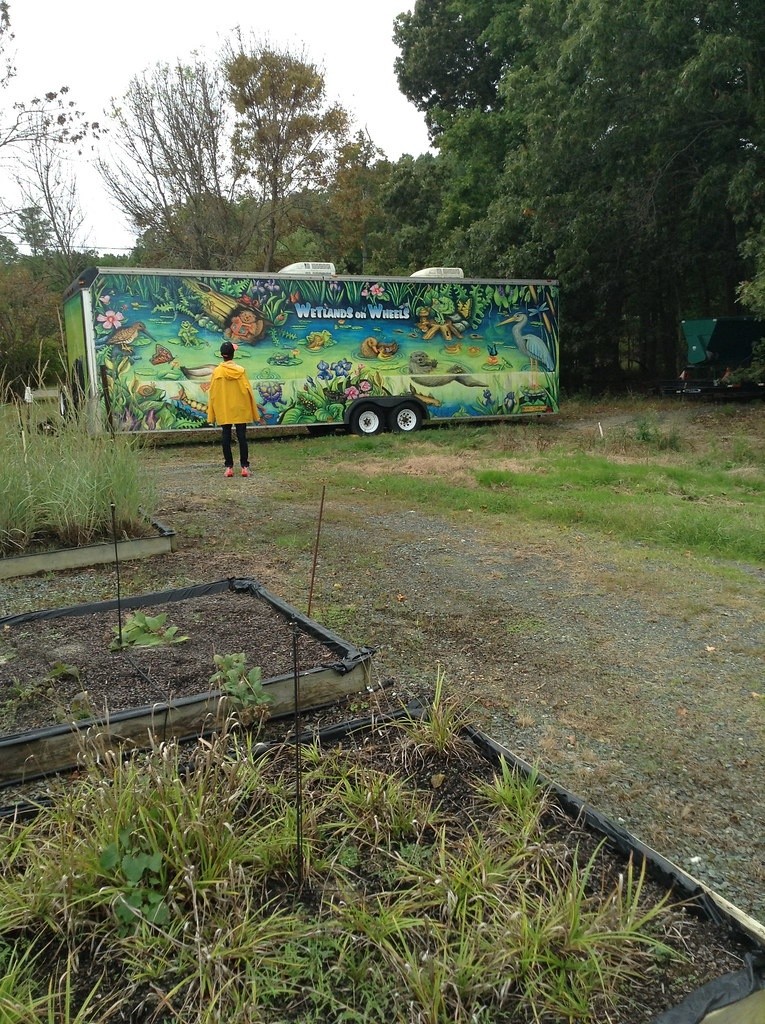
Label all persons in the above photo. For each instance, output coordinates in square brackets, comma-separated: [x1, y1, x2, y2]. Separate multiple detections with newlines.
[208, 342, 261, 477]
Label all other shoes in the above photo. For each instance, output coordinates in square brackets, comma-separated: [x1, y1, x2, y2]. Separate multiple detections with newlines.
[223, 467, 234, 477]
[241, 466, 254, 477]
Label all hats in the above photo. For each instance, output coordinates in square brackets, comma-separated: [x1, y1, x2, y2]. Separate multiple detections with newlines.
[219, 342, 234, 358]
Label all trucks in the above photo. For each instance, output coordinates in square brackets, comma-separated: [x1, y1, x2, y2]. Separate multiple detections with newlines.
[657, 316, 765, 403]
